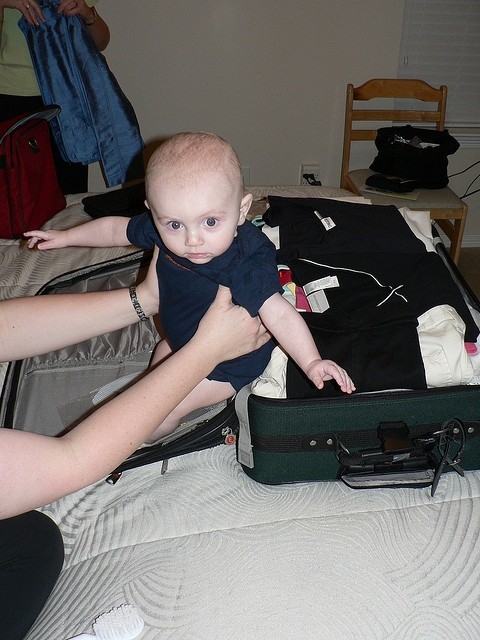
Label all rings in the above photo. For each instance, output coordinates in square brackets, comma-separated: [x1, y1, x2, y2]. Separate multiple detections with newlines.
[25, 5, 30, 9]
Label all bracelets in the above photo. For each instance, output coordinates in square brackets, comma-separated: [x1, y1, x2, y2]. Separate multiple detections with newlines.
[130, 286, 150, 322]
[85, 5, 97, 25]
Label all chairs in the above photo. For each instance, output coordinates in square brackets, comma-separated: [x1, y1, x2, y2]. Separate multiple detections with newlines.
[342, 78, 469, 267]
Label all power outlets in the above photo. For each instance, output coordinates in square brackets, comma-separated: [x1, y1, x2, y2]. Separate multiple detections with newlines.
[300, 163, 319, 186]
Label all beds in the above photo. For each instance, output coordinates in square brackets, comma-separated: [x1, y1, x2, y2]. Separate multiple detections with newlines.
[1, 185, 480, 640]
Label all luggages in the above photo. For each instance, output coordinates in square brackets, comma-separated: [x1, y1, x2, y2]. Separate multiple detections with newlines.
[0, 196, 480, 489]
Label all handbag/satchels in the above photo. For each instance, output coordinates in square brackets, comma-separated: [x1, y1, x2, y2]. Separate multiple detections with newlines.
[369, 126, 460, 189]
[0, 104, 67, 239]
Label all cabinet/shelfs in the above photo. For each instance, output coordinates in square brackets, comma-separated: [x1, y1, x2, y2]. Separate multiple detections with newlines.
[399, 1, 480, 248]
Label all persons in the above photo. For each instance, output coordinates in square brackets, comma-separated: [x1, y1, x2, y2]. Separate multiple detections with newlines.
[24, 132, 355, 444]
[0, 245, 271, 640]
[0, 0, 110, 193]
